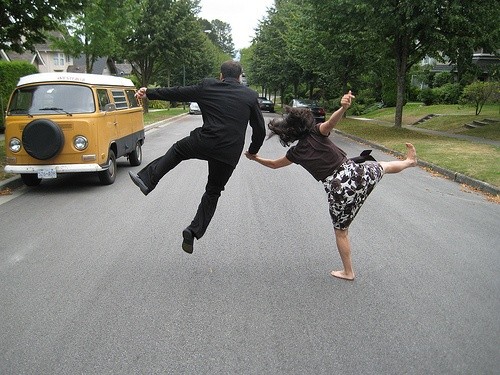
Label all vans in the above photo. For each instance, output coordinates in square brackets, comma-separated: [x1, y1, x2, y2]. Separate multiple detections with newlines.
[4, 72, 145, 187]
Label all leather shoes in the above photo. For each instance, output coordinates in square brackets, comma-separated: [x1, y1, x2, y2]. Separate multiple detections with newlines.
[182, 228, 194, 254]
[129, 170, 151, 196]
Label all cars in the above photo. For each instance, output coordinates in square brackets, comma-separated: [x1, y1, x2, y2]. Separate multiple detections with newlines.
[189, 102, 202, 115]
[257, 97, 274, 113]
[284, 99, 326, 124]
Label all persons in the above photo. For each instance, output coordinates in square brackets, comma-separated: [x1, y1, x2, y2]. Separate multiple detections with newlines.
[252, 90, 417, 280]
[128, 62, 266, 254]
